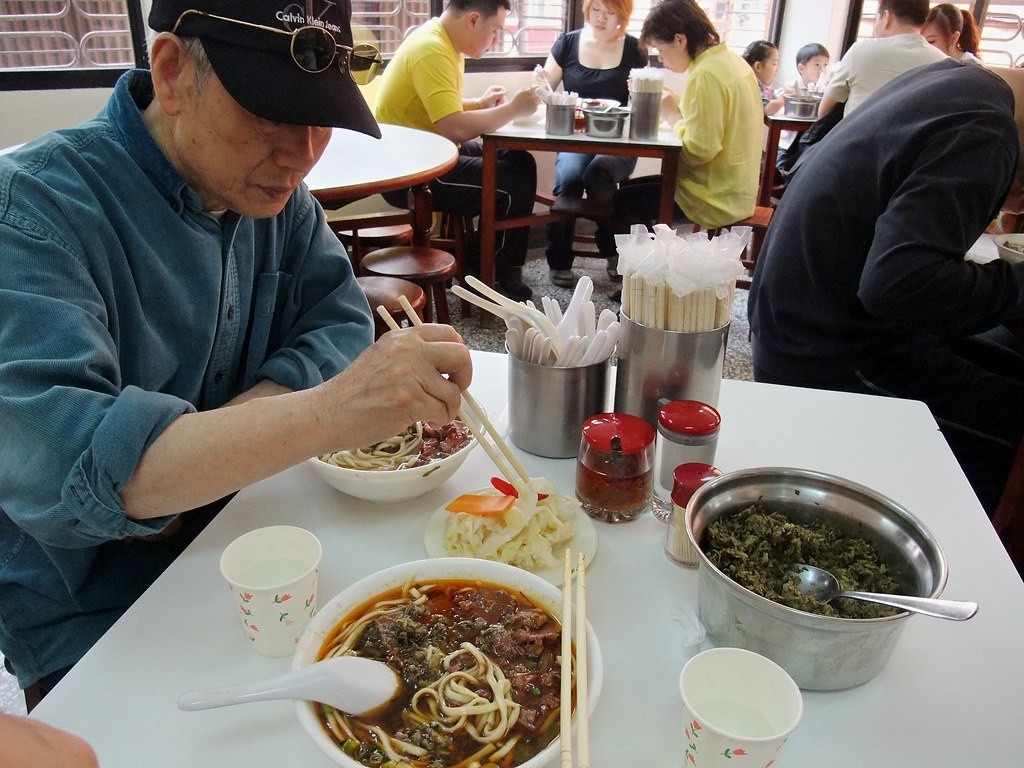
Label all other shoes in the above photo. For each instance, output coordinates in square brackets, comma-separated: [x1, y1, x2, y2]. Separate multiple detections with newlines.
[549, 267, 575, 287]
[496, 265, 532, 302]
[608, 289, 621, 302]
[606, 255, 623, 281]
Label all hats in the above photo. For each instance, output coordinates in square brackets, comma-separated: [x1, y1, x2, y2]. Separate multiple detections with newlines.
[148, 0, 382, 141]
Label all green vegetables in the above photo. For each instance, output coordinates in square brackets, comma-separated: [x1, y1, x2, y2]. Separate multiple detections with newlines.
[704, 489, 900, 619]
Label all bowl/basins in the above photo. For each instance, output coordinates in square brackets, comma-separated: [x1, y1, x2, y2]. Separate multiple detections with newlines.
[580, 99, 621, 113]
[992, 233, 1024, 265]
[308, 394, 489, 505]
[290, 555, 606, 768]
[583, 107, 630, 138]
[684, 466, 950, 691]
[783, 94, 821, 119]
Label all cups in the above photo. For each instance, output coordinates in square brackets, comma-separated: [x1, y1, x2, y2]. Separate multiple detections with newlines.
[678, 646, 804, 767]
[505, 340, 618, 460]
[219, 525, 323, 659]
[628, 90, 663, 143]
[614, 304, 732, 428]
[545, 104, 576, 135]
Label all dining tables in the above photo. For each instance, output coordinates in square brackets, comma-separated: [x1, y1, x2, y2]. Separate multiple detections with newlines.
[24, 348, 1024, 768]
[760, 113, 817, 208]
[479, 106, 684, 329]
[302, 122, 459, 326]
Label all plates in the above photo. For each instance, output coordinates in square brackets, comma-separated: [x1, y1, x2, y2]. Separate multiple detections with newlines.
[422, 486, 599, 587]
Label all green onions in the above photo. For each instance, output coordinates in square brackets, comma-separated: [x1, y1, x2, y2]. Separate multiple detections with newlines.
[323, 688, 541, 764]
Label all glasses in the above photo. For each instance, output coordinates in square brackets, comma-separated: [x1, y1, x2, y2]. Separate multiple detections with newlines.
[170, 10, 383, 86]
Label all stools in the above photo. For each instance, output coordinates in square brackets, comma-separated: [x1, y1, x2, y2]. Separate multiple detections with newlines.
[693, 203, 774, 290]
[337, 223, 457, 343]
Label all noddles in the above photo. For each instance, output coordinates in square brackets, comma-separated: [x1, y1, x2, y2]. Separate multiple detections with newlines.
[319, 419, 471, 470]
[318, 575, 575, 768]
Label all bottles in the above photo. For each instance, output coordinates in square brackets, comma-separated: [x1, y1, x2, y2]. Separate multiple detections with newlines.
[665, 462, 724, 568]
[575, 108, 585, 132]
[650, 400, 721, 523]
[573, 411, 656, 526]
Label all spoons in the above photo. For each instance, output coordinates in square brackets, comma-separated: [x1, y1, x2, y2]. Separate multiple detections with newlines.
[178, 655, 404, 718]
[451, 275, 621, 368]
[535, 87, 578, 105]
[788, 562, 979, 622]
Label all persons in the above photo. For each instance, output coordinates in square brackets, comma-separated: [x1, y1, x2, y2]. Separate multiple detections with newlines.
[603, 0, 764, 302]
[748, 52, 1024, 514]
[745, 0, 984, 204]
[535, 0, 650, 286]
[0, 712, 99, 768]
[373, 0, 543, 301]
[0, 0, 472, 713]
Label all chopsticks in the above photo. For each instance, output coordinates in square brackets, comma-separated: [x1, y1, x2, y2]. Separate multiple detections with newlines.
[376, 294, 530, 493]
[561, 547, 591, 767]
[621, 273, 738, 333]
[630, 77, 664, 93]
[537, 63, 553, 93]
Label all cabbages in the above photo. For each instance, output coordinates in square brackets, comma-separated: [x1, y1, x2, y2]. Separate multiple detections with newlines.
[446, 478, 574, 571]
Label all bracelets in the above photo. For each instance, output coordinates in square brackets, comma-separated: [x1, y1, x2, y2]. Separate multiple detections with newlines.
[473, 98, 480, 109]
